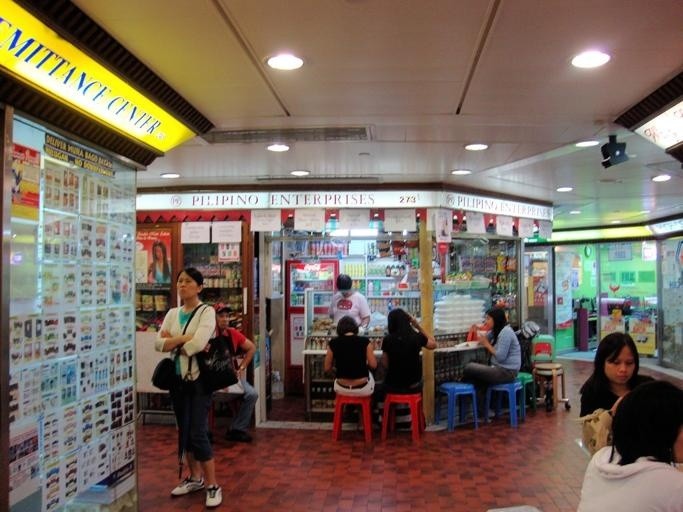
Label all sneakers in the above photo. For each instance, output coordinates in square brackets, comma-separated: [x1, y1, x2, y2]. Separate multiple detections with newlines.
[206, 483, 223, 507]
[169, 477, 205, 496]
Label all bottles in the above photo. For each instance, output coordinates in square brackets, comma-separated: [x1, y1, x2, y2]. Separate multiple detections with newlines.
[310, 359, 334, 379]
[290, 270, 332, 305]
[311, 385, 336, 407]
[344, 262, 406, 278]
[367, 298, 419, 322]
[311, 336, 383, 350]
[309, 239, 380, 255]
[433, 334, 489, 381]
[350, 280, 381, 295]
[203, 247, 243, 312]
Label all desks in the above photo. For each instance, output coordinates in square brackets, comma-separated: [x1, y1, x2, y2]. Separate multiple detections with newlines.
[588, 316, 597, 322]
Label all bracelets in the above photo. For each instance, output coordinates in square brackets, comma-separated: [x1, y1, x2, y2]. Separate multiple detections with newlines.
[239, 365, 245, 370]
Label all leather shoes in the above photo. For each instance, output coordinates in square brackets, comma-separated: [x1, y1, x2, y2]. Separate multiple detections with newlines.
[225, 429, 252, 443]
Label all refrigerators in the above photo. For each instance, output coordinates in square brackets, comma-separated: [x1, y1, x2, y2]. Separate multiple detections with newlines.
[283, 258, 339, 394]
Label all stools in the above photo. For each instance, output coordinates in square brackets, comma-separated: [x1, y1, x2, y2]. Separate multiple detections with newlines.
[532, 361, 571, 413]
[381, 392, 425, 441]
[435, 382, 480, 433]
[332, 393, 375, 442]
[530, 334, 556, 364]
[517, 371, 537, 421]
[482, 380, 526, 429]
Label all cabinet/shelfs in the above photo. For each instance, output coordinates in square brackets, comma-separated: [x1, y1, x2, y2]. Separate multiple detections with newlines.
[269, 232, 523, 422]
[135, 218, 255, 431]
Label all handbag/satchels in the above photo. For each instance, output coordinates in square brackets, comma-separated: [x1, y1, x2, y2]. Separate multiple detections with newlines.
[151, 357, 194, 402]
[581, 408, 613, 454]
[212, 359, 247, 401]
[193, 304, 238, 390]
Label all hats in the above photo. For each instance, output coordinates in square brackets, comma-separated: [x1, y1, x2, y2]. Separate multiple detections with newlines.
[213, 302, 233, 313]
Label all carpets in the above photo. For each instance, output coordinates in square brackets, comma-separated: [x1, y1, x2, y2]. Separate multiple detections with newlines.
[555, 346, 658, 368]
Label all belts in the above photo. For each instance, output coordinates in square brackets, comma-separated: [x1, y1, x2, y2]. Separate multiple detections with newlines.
[338, 382, 368, 389]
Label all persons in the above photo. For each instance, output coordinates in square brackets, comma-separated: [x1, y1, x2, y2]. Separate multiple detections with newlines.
[381, 308, 437, 394]
[579, 332, 656, 417]
[328, 274, 370, 327]
[155, 268, 223, 507]
[324, 316, 380, 424]
[576, 381, 683, 512]
[147, 241, 171, 284]
[462, 308, 521, 422]
[213, 303, 258, 441]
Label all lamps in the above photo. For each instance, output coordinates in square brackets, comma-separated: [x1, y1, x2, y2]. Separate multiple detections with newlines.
[601, 134, 629, 168]
